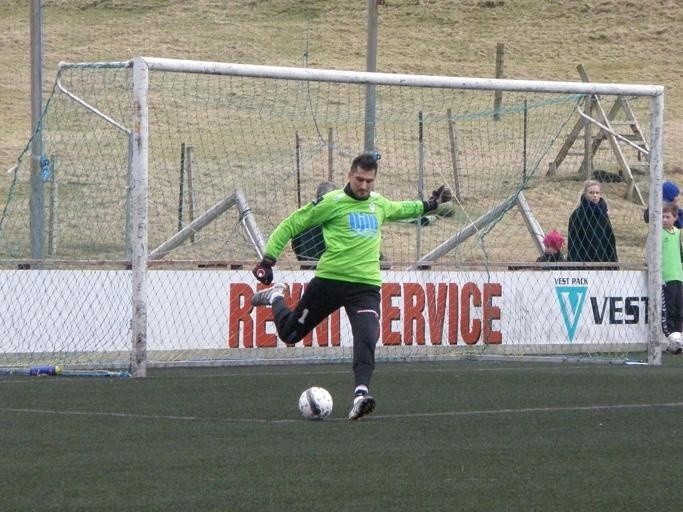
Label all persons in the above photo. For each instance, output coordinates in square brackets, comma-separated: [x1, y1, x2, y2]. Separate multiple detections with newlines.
[292, 181, 390, 269]
[567, 179, 621, 270]
[534, 228, 567, 270]
[249, 152, 452, 420]
[644, 181, 683, 228]
[648, 202, 683, 353]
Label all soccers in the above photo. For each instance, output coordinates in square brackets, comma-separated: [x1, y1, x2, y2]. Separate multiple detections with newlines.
[299, 386, 333, 420]
[668, 332, 683, 353]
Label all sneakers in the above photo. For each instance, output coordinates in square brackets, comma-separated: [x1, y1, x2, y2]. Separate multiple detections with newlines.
[250, 283, 286, 306]
[348, 396, 376, 419]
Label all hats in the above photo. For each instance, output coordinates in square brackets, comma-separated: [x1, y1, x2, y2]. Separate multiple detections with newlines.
[663, 182, 680, 202]
[542, 228, 566, 252]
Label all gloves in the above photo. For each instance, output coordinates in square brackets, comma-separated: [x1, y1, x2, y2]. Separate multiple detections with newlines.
[421, 183, 451, 217]
[252, 254, 276, 285]
